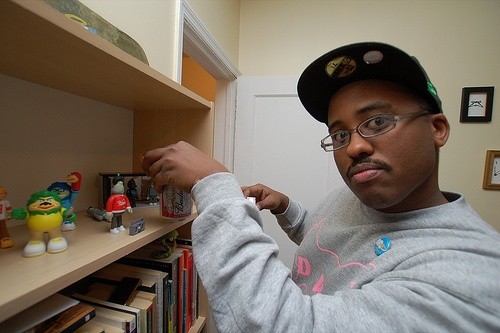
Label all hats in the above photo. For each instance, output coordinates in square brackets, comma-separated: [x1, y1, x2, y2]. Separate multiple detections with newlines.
[298, 41, 443, 123]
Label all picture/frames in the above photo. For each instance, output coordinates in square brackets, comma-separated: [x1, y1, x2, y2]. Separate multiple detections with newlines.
[459, 86, 494, 123]
[481, 149, 500, 191]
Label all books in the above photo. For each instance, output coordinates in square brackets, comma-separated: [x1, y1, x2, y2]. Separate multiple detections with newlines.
[0, 233, 200, 333]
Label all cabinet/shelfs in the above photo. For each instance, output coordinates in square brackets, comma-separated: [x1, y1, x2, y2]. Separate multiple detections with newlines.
[0, 0, 214, 333]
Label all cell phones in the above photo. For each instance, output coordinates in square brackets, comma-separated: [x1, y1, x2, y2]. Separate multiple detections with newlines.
[108, 276, 143, 306]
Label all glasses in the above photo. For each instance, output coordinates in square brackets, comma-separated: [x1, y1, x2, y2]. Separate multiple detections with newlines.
[320, 109, 430, 152]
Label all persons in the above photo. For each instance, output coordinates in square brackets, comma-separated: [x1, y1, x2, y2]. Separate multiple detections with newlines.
[141, 41, 500, 333]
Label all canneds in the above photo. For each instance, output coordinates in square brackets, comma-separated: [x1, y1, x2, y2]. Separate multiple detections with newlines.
[159, 185, 194, 219]
